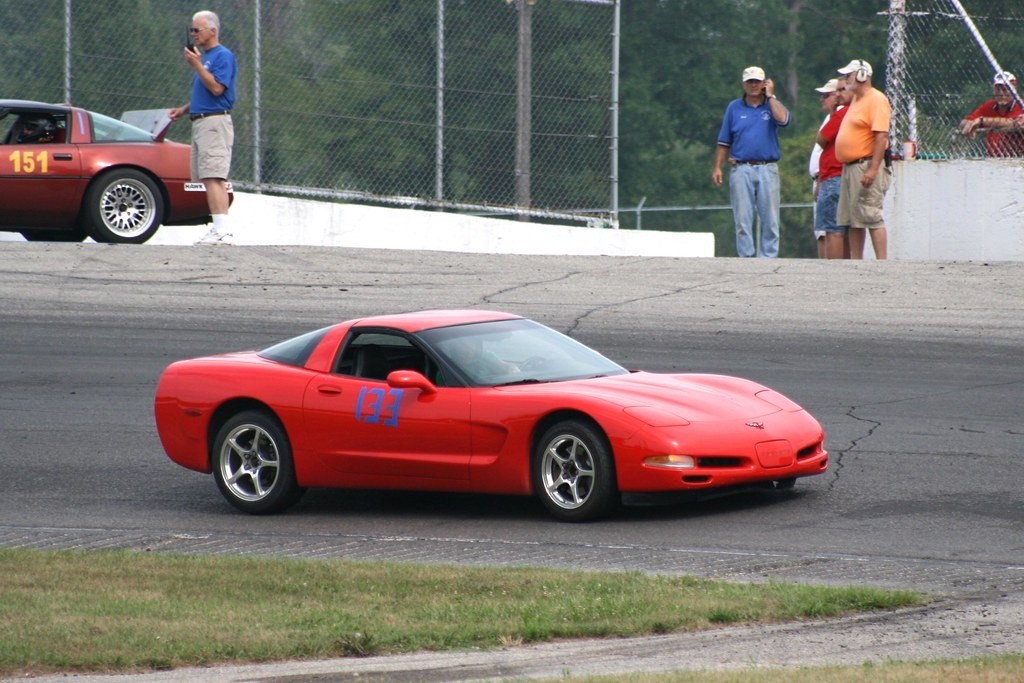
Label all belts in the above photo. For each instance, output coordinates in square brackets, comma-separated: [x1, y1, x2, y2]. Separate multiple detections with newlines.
[190, 110, 231, 121]
[813, 176, 818, 180]
[738, 160, 776, 165]
[845, 157, 872, 165]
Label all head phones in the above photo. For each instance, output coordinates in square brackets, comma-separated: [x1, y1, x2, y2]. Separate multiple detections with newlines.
[856, 58, 867, 83]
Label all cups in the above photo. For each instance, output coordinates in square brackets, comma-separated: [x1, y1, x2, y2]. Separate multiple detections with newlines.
[903, 141, 916, 160]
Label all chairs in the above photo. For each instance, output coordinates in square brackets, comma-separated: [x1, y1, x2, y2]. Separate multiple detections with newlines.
[350, 343, 391, 379]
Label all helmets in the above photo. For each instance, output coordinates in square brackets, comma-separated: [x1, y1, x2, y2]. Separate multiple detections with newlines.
[15, 112, 56, 144]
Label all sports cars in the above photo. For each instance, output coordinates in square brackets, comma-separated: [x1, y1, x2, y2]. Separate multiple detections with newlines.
[0, 98, 234, 244]
[153, 307, 832, 523]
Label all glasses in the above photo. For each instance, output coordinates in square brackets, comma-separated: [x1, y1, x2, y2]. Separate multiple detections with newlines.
[820, 94, 838, 98]
[190, 27, 211, 33]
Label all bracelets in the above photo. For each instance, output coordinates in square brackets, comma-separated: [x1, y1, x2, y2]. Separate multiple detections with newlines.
[980, 116, 983, 126]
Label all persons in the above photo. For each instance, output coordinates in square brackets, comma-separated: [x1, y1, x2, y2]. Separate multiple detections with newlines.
[808, 59, 892, 260]
[960, 71, 1024, 159]
[712, 66, 791, 258]
[168, 10, 238, 246]
[16, 112, 58, 144]
[436, 336, 520, 387]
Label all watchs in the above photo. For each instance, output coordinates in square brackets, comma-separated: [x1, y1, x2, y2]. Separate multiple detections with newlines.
[767, 94, 776, 101]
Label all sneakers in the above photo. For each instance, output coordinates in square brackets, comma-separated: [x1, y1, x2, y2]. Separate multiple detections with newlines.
[193, 227, 233, 246]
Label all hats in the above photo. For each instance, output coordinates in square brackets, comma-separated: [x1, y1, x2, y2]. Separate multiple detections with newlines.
[815, 79, 839, 93]
[994, 72, 1017, 87]
[837, 60, 873, 77]
[742, 67, 765, 82]
